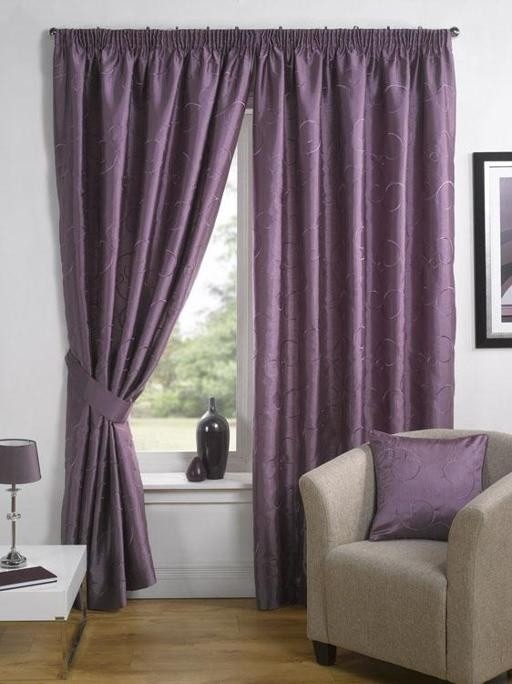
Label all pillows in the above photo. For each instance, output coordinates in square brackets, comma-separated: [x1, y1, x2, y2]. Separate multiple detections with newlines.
[359, 429, 492, 541]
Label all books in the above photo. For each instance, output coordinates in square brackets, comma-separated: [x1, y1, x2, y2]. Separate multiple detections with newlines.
[0, 566, 58, 590]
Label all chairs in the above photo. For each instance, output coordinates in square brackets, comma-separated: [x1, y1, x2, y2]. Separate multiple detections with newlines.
[299, 421, 511, 683]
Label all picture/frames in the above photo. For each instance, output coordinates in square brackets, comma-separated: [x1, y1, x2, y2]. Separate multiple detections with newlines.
[471, 149, 512, 346]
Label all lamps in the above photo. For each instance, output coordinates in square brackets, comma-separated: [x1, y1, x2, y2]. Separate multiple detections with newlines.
[3, 441, 46, 565]
